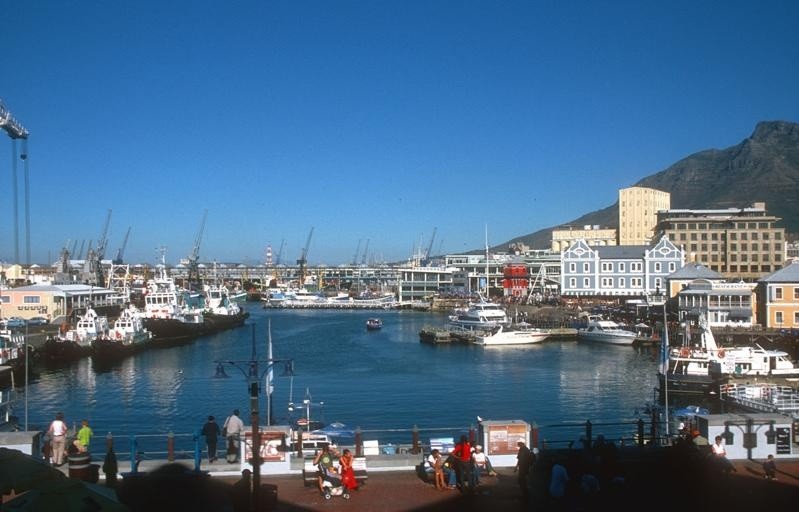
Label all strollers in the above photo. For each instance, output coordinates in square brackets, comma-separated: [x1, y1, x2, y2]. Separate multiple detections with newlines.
[317, 462, 351, 500]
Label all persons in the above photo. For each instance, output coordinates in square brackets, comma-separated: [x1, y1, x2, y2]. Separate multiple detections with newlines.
[41, 413, 93, 467]
[234, 469, 254, 496]
[223, 409, 244, 463]
[764, 455, 776, 480]
[201, 415, 220, 463]
[425, 435, 497, 491]
[313, 443, 358, 498]
[515, 433, 623, 499]
[683, 431, 733, 474]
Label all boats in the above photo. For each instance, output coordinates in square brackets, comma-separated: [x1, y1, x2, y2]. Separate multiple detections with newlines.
[0, 254, 250, 386]
[447, 326, 483, 343]
[475, 309, 551, 347]
[366, 318, 383, 330]
[420, 325, 452, 346]
[261, 286, 395, 310]
[578, 320, 637, 346]
[659, 309, 799, 397]
[449, 293, 512, 328]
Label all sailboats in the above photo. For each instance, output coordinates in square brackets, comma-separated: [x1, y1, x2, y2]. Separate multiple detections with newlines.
[266, 316, 338, 451]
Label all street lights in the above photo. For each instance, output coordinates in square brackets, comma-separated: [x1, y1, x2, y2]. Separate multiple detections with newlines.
[214, 323, 296, 512]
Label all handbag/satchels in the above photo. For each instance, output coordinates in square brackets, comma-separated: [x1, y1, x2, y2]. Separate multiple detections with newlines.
[222, 428, 226, 436]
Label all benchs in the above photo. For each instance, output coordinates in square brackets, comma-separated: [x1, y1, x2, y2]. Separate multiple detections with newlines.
[304, 456, 366, 490]
[422, 449, 489, 482]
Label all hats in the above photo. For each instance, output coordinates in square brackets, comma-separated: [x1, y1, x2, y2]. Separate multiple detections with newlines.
[516, 438, 525, 443]
